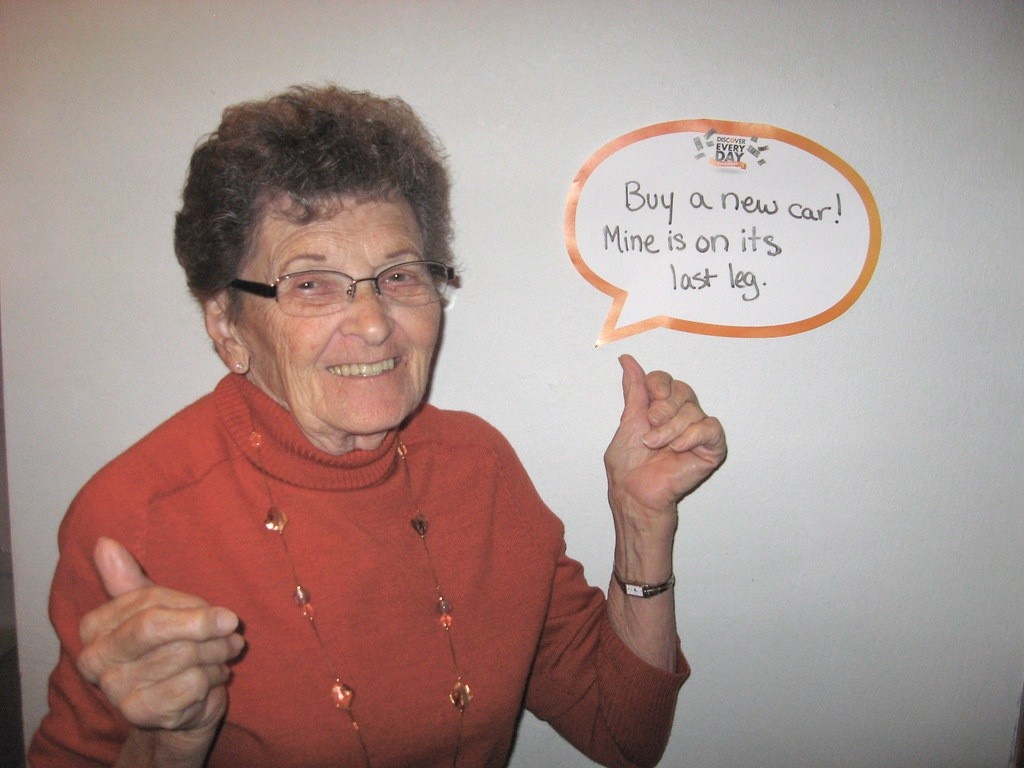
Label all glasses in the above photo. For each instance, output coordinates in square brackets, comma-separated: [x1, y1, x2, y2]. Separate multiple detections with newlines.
[227, 261, 455, 319]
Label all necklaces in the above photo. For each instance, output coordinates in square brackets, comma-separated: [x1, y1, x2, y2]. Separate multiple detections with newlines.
[228, 375, 475, 766]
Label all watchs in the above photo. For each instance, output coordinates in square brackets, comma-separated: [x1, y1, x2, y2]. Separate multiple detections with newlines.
[614, 567, 676, 598]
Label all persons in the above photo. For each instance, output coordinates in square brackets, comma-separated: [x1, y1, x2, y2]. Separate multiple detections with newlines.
[26, 87, 727, 768]
